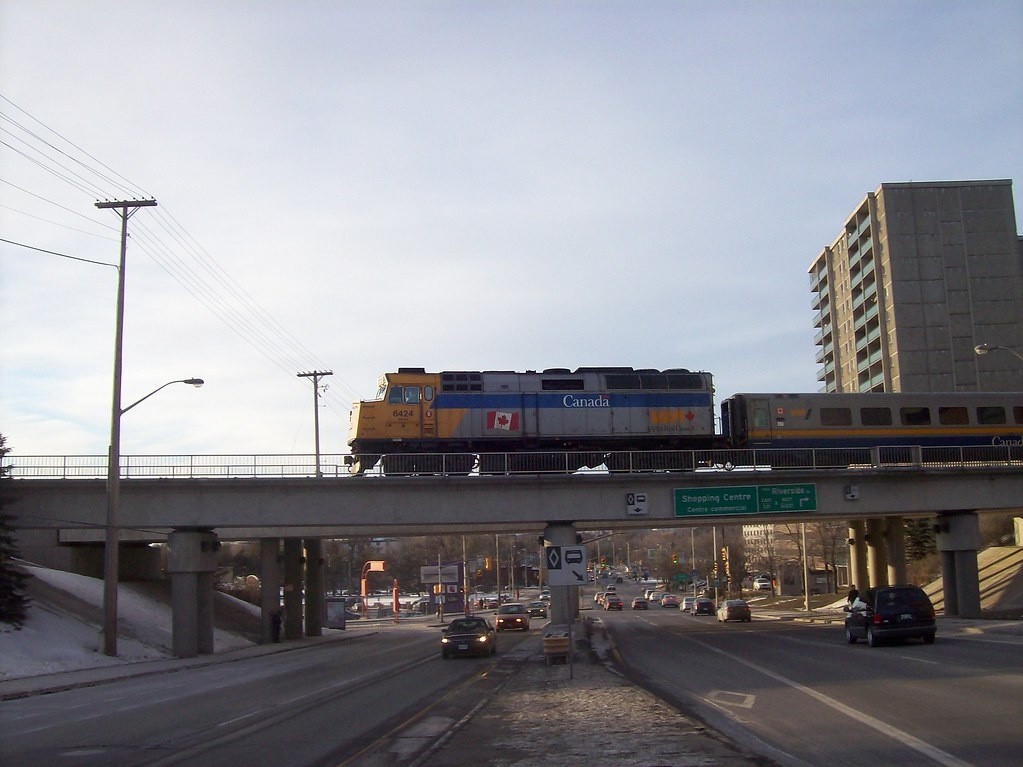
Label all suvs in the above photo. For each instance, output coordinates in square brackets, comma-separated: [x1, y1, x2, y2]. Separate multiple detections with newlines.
[753, 578, 771, 591]
[843, 584, 938, 646]
[596, 592, 616, 605]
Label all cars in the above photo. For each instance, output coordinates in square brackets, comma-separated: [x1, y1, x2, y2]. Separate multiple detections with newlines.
[587, 563, 649, 590]
[644, 589, 658, 600]
[662, 595, 679, 607]
[689, 598, 716, 616]
[658, 592, 673, 603]
[527, 601, 548, 620]
[353, 602, 366, 612]
[472, 600, 499, 609]
[680, 597, 698, 611]
[631, 597, 648, 610]
[604, 595, 624, 611]
[648, 592, 661, 602]
[594, 592, 604, 601]
[538, 590, 552, 601]
[441, 616, 497, 659]
[548, 603, 553, 609]
[716, 600, 751, 622]
[493, 603, 531, 631]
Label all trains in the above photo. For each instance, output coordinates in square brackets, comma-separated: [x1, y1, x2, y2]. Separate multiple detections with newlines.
[343, 366, 1023, 472]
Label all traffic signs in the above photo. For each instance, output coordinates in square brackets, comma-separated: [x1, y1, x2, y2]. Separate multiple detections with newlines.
[545, 545, 586, 586]
[625, 493, 649, 515]
[672, 482, 820, 518]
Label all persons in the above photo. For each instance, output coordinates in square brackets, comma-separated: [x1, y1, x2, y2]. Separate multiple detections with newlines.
[405, 388, 419, 404]
[847, 585, 859, 605]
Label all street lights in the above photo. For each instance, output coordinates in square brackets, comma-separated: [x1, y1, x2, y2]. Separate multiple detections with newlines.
[973, 342, 1023, 366]
[94, 195, 205, 660]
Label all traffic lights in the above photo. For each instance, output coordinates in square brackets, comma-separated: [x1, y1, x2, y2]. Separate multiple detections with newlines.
[721, 547, 726, 561]
[600, 559, 606, 568]
[673, 555, 678, 564]
[724, 561, 730, 574]
[714, 562, 718, 571]
